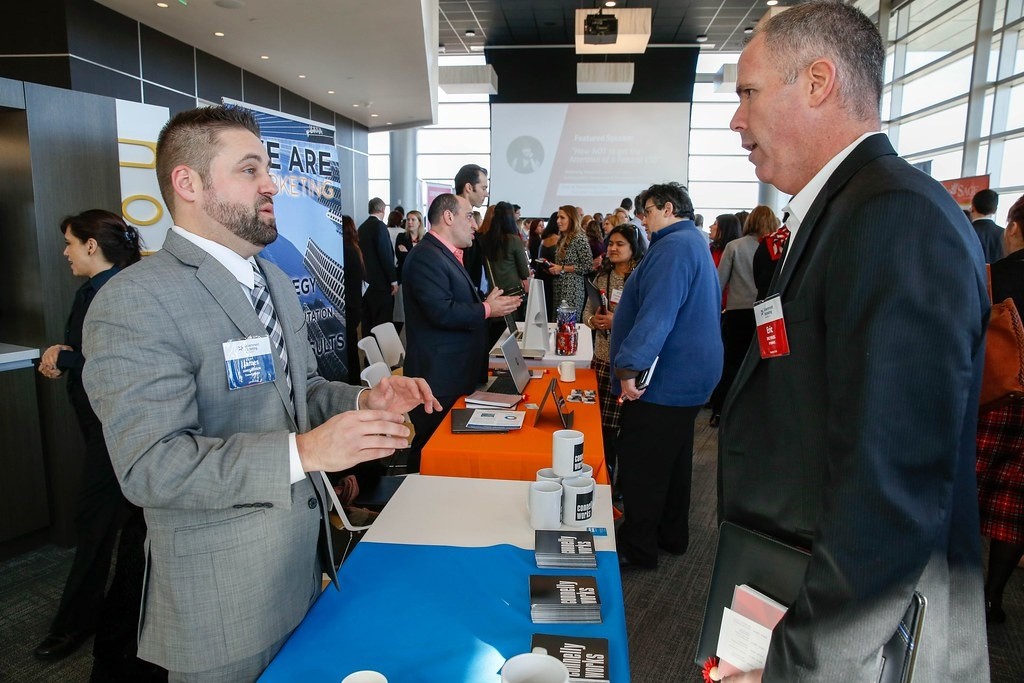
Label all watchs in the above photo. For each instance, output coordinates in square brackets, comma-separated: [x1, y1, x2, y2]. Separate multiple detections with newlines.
[561, 265, 565, 274]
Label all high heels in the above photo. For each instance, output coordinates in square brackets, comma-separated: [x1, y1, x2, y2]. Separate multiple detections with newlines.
[710, 411, 720, 428]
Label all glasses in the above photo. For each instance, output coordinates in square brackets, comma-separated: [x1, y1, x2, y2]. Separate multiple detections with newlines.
[643, 204, 663, 217]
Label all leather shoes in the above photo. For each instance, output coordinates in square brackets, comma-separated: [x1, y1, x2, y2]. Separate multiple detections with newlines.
[34, 629, 93, 659]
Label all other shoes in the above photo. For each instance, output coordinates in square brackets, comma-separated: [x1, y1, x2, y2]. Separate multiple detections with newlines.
[618, 553, 655, 568]
[985, 599, 1007, 622]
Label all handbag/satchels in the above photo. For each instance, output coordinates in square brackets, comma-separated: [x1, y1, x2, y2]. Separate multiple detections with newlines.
[979, 296, 1024, 405]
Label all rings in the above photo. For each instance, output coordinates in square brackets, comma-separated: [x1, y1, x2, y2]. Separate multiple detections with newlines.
[601, 324, 604, 328]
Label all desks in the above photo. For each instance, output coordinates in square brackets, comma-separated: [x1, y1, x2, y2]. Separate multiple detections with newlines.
[253, 471, 631, 682]
[419, 365, 608, 486]
[489, 313, 594, 369]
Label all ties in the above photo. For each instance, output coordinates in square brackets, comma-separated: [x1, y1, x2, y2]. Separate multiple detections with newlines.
[249, 264, 295, 414]
[454, 250, 464, 267]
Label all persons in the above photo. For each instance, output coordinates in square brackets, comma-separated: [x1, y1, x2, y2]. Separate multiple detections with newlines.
[582, 223, 648, 500]
[478, 201, 531, 323]
[708, 205, 779, 427]
[341, 198, 425, 372]
[462, 211, 484, 291]
[621, 198, 634, 221]
[608, 185, 724, 573]
[718, 1, 994, 683]
[33, 210, 142, 665]
[454, 163, 490, 210]
[403, 193, 524, 417]
[81, 105, 443, 683]
[963, 186, 1024, 611]
[517, 205, 630, 323]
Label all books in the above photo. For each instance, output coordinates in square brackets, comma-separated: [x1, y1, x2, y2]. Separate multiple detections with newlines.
[695, 519, 925, 683]
[616, 355, 660, 406]
[587, 277, 608, 341]
[450, 390, 526, 434]
[535, 257, 562, 276]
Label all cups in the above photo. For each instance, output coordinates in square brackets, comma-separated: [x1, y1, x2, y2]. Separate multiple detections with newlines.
[342, 670, 388, 683]
[528, 430, 596, 530]
[500, 653, 569, 683]
[557, 361, 576, 382]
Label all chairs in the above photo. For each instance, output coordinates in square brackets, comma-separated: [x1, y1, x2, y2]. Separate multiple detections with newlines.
[319, 472, 377, 572]
[358, 321, 416, 443]
